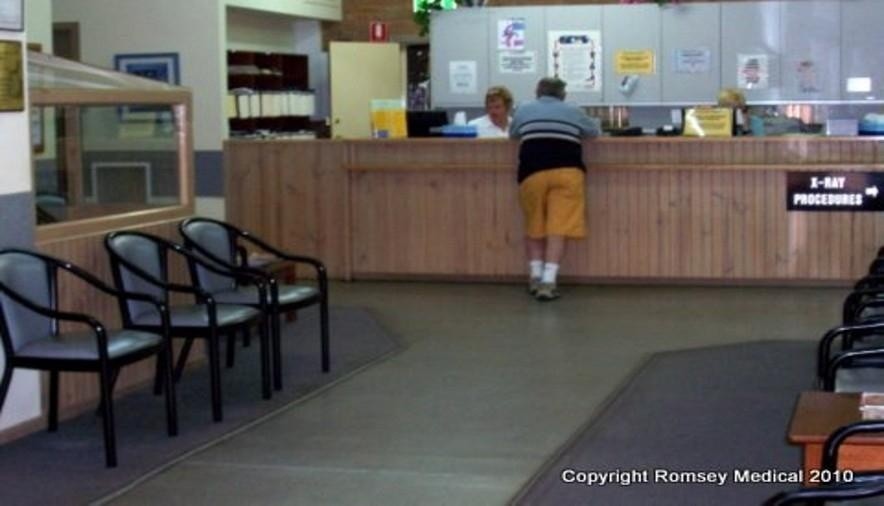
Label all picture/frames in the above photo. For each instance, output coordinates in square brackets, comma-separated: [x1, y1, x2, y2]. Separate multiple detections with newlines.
[26, 39, 48, 156]
[113, 51, 181, 127]
[1, 41, 26, 115]
[1, 1, 27, 33]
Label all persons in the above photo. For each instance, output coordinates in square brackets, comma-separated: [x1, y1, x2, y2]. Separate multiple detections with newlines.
[466, 83, 513, 138]
[716, 86, 766, 138]
[507, 74, 600, 301]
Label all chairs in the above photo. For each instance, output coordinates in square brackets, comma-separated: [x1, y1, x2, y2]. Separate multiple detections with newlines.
[101, 227, 274, 428]
[178, 216, 333, 394]
[760, 243, 884, 506]
[1, 248, 182, 471]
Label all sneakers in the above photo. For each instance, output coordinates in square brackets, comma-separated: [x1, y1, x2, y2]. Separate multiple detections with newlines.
[535, 283, 561, 300]
[528, 277, 540, 294]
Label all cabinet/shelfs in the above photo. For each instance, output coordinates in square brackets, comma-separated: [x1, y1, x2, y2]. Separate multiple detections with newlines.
[546, 3, 664, 110]
[662, 3, 783, 109]
[783, 3, 883, 109]
[429, 3, 546, 116]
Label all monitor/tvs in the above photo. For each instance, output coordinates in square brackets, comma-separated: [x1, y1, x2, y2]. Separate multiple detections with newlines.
[406, 110, 446, 139]
